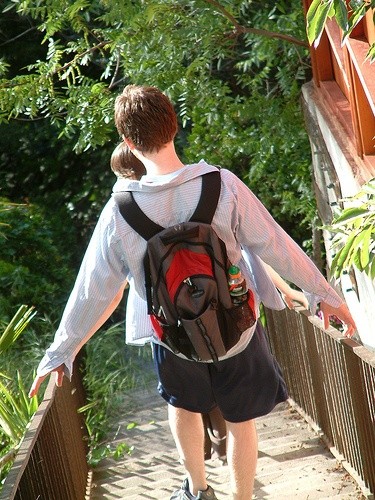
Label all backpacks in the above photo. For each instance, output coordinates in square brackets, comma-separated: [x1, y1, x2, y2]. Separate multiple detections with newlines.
[112, 172, 256, 360]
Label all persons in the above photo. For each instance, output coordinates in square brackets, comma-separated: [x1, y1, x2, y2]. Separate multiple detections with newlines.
[25, 85, 358, 500]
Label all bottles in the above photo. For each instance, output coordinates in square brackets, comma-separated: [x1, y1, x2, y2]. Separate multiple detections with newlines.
[226, 266, 254, 331]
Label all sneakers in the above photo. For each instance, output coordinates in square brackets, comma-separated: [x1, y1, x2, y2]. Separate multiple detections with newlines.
[170, 476, 217, 500]
